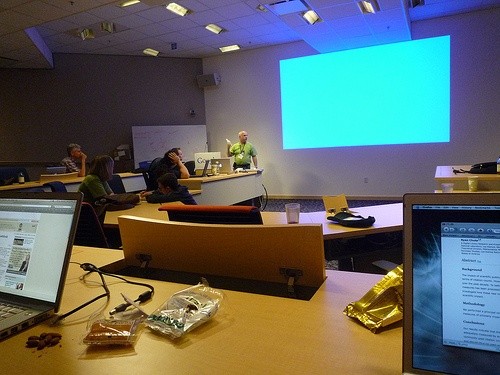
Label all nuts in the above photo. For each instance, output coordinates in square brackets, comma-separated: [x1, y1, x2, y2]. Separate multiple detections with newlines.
[26, 331, 62, 348]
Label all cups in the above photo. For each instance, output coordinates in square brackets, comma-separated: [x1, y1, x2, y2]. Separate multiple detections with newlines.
[211, 165, 218, 174]
[285, 203, 300, 224]
[441, 183, 454, 192]
[467, 176, 479, 192]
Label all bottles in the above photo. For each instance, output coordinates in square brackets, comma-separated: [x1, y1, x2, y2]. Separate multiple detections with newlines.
[18, 171, 24, 184]
[496, 156, 500, 175]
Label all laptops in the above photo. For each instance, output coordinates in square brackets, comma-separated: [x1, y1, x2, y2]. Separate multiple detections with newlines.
[195, 151, 221, 170]
[402, 192, 500, 375]
[210, 159, 232, 174]
[0, 190, 83, 340]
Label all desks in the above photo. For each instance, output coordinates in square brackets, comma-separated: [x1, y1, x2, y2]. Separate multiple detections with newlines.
[433, 165, 500, 193]
[0, 171, 402, 375]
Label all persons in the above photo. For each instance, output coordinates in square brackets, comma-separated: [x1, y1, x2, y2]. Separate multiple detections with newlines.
[155, 148, 189, 191]
[60, 143, 87, 177]
[77, 154, 154, 204]
[227, 131, 257, 170]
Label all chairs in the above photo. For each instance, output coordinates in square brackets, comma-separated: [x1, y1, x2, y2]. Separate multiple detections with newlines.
[43, 181, 67, 192]
[107, 175, 125, 194]
[0, 167, 30, 186]
[74, 203, 108, 248]
[321, 193, 347, 211]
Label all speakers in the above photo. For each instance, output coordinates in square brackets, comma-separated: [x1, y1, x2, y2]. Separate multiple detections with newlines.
[196, 73, 221, 88]
